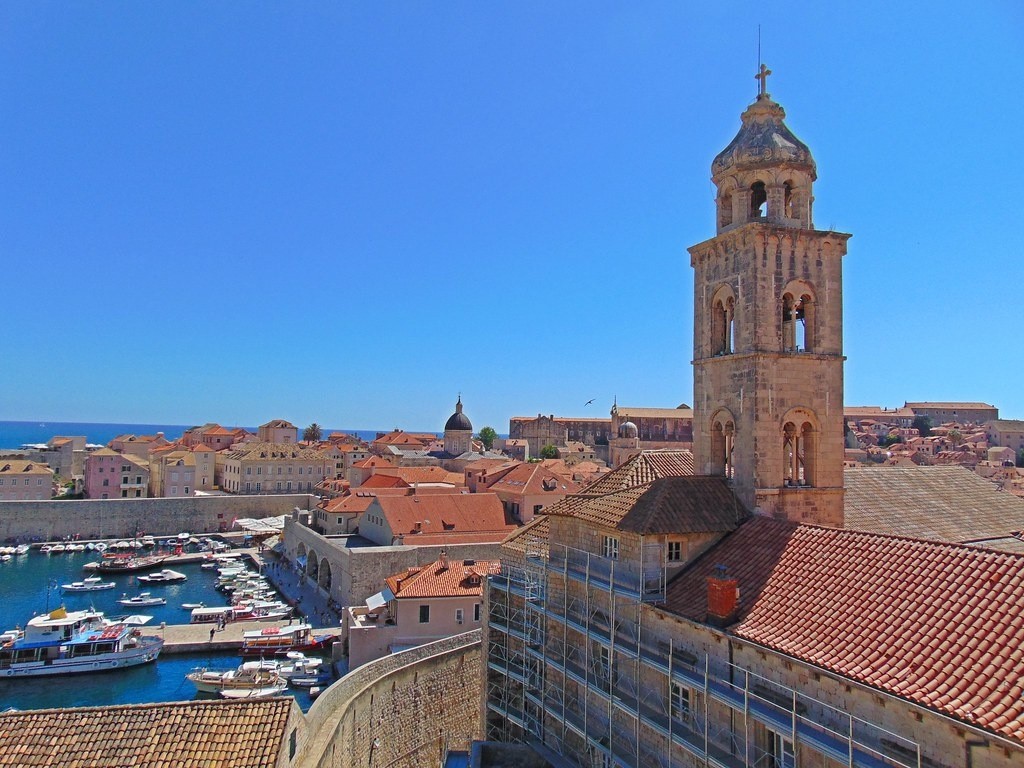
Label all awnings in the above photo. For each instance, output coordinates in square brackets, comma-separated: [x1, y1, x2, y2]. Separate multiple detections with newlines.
[296, 555, 307, 566]
[262, 535, 284, 552]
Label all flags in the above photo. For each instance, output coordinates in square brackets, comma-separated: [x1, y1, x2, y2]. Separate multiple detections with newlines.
[230, 516, 238, 529]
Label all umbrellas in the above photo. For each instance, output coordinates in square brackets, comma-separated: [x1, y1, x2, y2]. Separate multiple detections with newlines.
[243, 535, 253, 539]
[122, 615, 153, 629]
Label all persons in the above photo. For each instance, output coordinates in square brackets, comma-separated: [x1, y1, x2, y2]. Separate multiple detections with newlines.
[295, 565, 299, 574]
[300, 570, 306, 585]
[299, 613, 309, 625]
[289, 616, 293, 625]
[217, 616, 226, 630]
[272, 562, 279, 569]
[194, 615, 214, 621]
[186, 544, 189, 554]
[211, 548, 216, 554]
[312, 569, 318, 579]
[210, 628, 215, 642]
[258, 609, 269, 616]
[227, 612, 232, 623]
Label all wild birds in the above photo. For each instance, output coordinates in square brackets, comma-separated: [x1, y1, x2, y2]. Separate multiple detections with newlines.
[584, 399, 596, 406]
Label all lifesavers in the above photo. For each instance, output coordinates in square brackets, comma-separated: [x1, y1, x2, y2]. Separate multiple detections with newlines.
[233, 606, 245, 609]
[261, 628, 279, 635]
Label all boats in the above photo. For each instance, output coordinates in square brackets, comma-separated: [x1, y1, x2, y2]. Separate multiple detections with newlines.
[0, 534, 333, 700]
